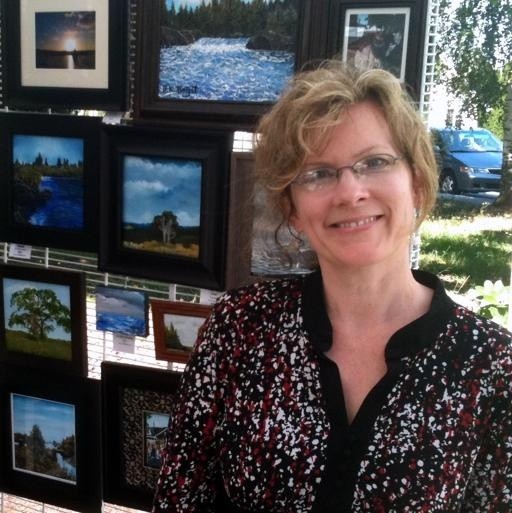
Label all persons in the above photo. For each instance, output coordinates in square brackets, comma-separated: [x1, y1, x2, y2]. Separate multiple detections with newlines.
[147, 58, 512, 513]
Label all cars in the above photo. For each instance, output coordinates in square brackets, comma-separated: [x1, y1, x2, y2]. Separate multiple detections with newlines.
[428, 125, 503, 197]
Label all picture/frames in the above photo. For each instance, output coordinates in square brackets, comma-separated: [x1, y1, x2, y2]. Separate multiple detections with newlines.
[1, 356, 105, 513]
[1, 1, 133, 116]
[94, 283, 151, 339]
[233, 146, 319, 294]
[325, 2, 429, 113]
[1, 257, 90, 385]
[130, 0, 332, 125]
[102, 120, 235, 293]
[99, 358, 185, 513]
[149, 298, 212, 366]
[2, 111, 104, 258]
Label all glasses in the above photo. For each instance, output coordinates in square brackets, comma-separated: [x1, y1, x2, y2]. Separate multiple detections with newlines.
[290, 153, 407, 192]
